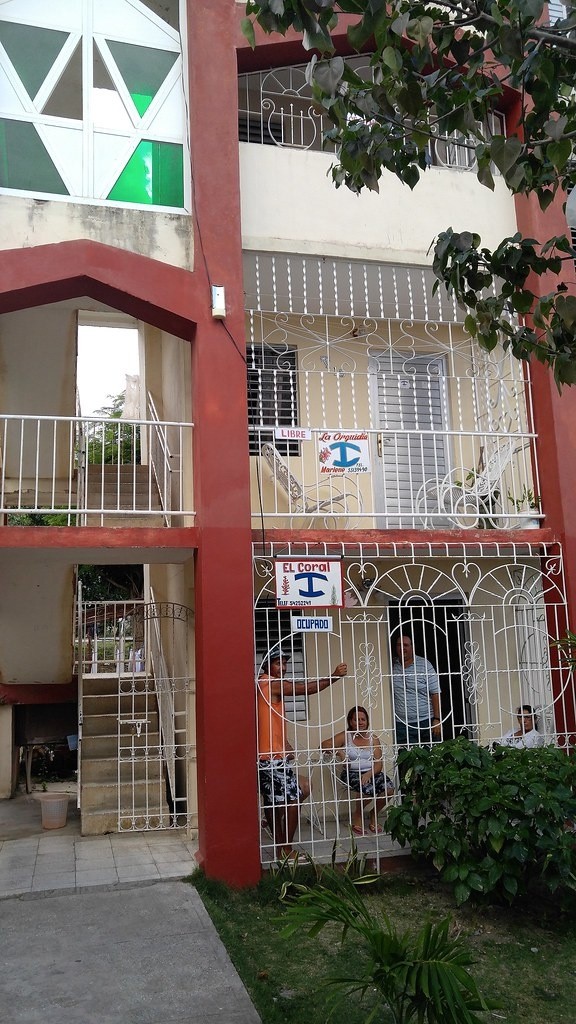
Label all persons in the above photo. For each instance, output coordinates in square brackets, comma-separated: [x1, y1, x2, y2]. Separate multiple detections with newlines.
[318, 705, 395, 836]
[392, 634, 442, 805]
[499, 705, 545, 750]
[256, 645, 347, 863]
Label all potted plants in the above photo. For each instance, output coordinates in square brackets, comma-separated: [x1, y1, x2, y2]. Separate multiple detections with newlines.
[504, 487, 542, 529]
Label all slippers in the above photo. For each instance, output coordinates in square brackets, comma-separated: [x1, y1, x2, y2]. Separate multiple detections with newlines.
[369, 823, 382, 833]
[352, 824, 369, 837]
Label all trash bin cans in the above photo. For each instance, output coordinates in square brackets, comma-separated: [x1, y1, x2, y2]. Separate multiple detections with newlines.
[39, 793, 70, 829]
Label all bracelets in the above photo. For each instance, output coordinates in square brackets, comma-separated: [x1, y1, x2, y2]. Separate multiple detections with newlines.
[432, 716, 440, 722]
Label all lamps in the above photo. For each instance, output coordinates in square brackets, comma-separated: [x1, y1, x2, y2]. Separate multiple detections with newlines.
[211, 284, 227, 319]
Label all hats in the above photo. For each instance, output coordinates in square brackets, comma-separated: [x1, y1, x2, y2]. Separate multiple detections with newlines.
[261, 645, 292, 670]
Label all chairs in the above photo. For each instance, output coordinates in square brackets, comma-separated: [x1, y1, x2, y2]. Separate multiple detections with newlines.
[415, 441, 518, 530]
[320, 738, 398, 826]
[533, 703, 554, 744]
[262, 442, 364, 530]
[261, 736, 323, 840]
